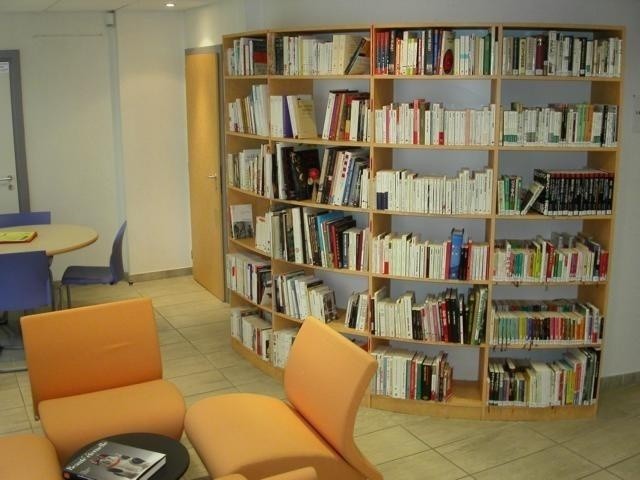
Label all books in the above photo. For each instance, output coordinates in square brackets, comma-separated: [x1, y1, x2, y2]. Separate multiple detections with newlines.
[229, 203, 368, 272]
[499, 101, 617, 147]
[370, 285, 488, 345]
[373, 166, 494, 215]
[226, 31, 369, 78]
[369, 344, 453, 402]
[63, 440, 166, 480]
[489, 299, 604, 345]
[226, 253, 370, 333]
[226, 140, 372, 208]
[492, 232, 609, 283]
[486, 348, 601, 408]
[375, 31, 491, 76]
[348, 338, 368, 353]
[496, 168, 615, 216]
[230, 306, 298, 370]
[502, 31, 621, 77]
[227, 86, 371, 143]
[372, 228, 490, 281]
[375, 98, 496, 146]
[0, 231, 38, 242]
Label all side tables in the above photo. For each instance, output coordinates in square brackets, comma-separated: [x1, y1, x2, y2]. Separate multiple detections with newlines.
[64, 432, 190, 480]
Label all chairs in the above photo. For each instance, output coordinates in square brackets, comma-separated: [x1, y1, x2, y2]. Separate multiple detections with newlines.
[0, 434, 64, 480]
[183, 316, 385, 480]
[60, 221, 131, 310]
[0, 250, 57, 372]
[0, 212, 53, 224]
[19, 294, 186, 467]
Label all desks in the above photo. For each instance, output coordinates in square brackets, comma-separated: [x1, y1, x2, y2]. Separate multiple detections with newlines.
[0, 223, 98, 323]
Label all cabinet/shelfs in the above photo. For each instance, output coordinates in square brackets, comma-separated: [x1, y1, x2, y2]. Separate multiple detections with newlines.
[222, 23, 627, 422]
